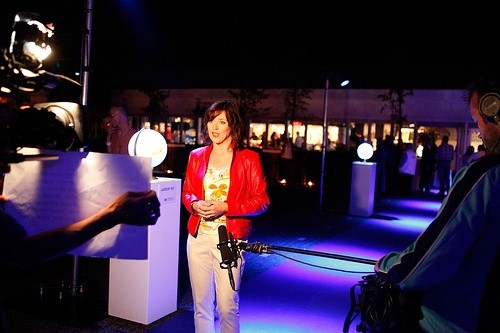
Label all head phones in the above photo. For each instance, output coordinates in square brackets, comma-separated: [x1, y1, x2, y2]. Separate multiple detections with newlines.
[478, 92, 500, 128]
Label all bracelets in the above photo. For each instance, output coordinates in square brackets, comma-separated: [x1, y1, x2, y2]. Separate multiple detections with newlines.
[190, 200, 196, 207]
[106, 141, 112, 147]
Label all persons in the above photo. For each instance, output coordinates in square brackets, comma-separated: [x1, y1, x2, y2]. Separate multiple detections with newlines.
[415, 136, 434, 195]
[106, 107, 137, 156]
[342, 130, 404, 197]
[374, 75, 500, 333]
[182, 98, 269, 333]
[259, 131, 305, 175]
[0, 112, 161, 333]
[460, 144, 475, 163]
[433, 135, 455, 198]
[102, 116, 113, 153]
[399, 142, 416, 196]
[474, 144, 487, 159]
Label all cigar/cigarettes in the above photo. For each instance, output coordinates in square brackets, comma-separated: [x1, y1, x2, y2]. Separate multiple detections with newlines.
[107, 123, 111, 126]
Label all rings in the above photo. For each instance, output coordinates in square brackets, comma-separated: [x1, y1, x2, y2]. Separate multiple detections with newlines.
[204, 207, 208, 212]
[145, 202, 155, 218]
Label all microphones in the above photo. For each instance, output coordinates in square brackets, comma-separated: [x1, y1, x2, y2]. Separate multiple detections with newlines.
[218, 225, 235, 290]
